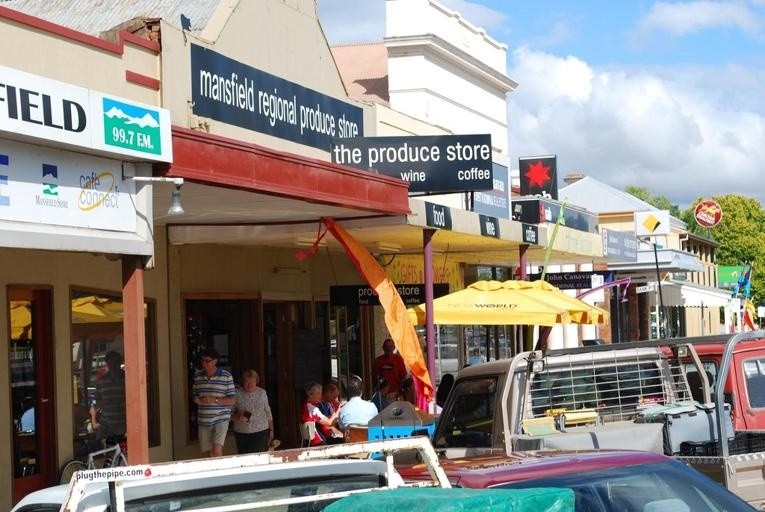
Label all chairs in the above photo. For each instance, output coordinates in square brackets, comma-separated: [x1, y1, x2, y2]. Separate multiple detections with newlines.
[643, 497, 693, 512]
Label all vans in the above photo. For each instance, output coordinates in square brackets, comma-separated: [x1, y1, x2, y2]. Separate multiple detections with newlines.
[658, 329, 765, 432]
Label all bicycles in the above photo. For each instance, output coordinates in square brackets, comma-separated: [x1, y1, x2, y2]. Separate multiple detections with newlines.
[57, 430, 127, 484]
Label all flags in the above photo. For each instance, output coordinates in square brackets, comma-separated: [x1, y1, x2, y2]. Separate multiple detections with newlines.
[732, 269, 746, 296]
[741, 266, 755, 298]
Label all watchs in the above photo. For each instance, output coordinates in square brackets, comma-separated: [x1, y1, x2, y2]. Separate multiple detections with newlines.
[215, 396, 219, 404]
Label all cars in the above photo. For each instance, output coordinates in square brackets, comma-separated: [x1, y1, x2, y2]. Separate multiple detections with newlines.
[9, 434, 765, 512]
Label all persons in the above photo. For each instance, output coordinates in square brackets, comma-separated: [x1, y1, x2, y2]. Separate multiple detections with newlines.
[231, 369, 275, 454]
[297, 376, 392, 446]
[372, 338, 408, 399]
[192, 350, 236, 458]
[18, 408, 36, 433]
[87, 405, 104, 430]
[95, 350, 126, 441]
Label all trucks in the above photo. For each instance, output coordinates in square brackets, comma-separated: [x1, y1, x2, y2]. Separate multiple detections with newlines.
[423, 349, 765, 512]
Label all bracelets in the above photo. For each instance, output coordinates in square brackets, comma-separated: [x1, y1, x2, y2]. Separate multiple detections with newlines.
[269, 429, 274, 431]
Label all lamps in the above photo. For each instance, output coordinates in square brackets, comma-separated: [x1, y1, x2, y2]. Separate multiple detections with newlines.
[123, 174, 187, 219]
